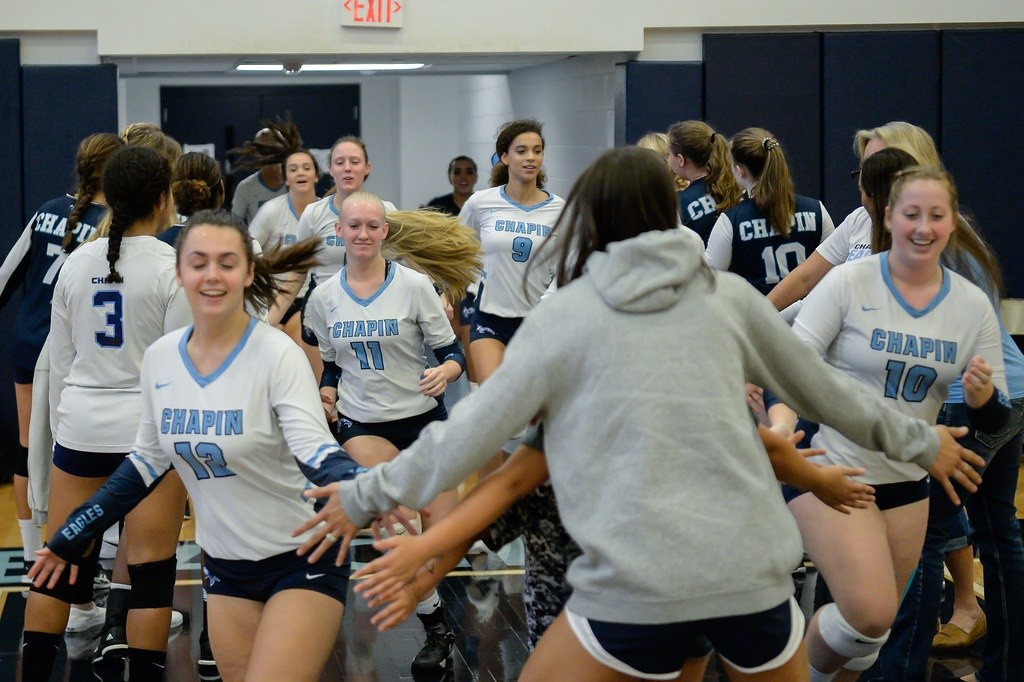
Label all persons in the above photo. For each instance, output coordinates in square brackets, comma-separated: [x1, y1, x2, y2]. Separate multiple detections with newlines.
[0, 118, 1024, 682]
[292, 145, 986, 682]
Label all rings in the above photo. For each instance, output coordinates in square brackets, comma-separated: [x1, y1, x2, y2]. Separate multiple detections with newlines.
[327, 533, 337, 544]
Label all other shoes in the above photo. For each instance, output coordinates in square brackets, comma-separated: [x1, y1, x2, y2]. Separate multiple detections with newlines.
[65, 601, 107, 635]
[21, 560, 37, 585]
[931, 608, 988, 647]
[170, 612, 184, 630]
[93, 566, 115, 587]
[198, 631, 221, 681]
[91, 628, 128, 664]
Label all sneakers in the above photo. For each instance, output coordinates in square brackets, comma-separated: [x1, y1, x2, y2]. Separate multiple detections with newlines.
[411, 624, 457, 672]
